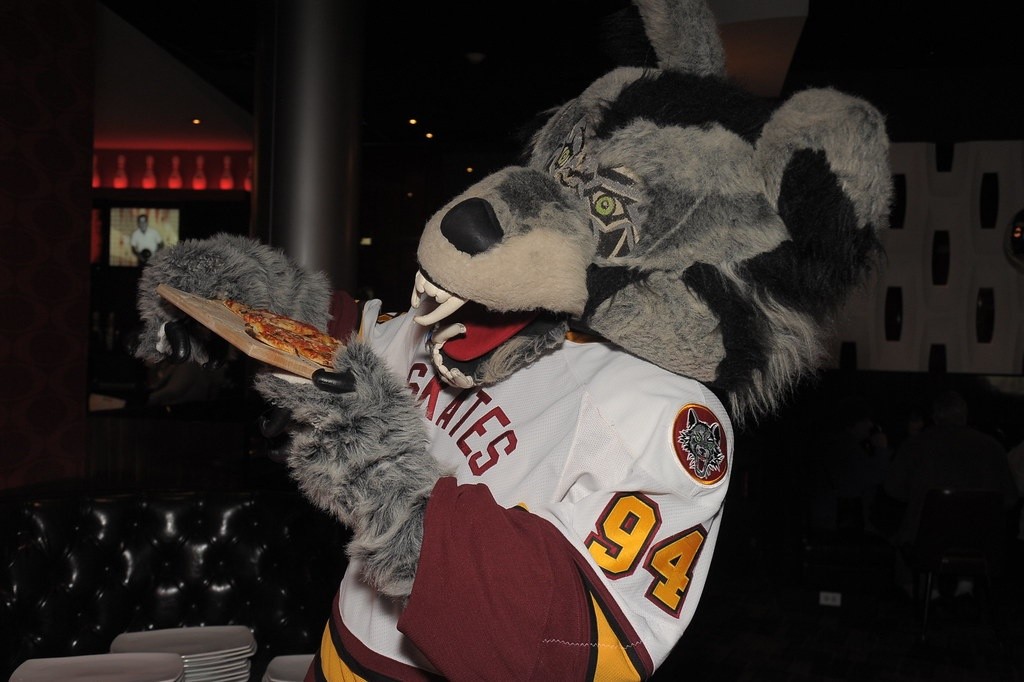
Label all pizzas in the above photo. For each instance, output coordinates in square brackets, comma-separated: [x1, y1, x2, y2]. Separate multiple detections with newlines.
[223, 296, 345, 373]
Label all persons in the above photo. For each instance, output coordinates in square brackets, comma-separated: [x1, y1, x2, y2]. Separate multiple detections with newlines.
[131, 215, 163, 260]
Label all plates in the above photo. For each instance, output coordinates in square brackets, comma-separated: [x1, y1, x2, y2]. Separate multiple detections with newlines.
[9, 652, 186, 682]
[110, 625, 256, 682]
[262, 654, 317, 682]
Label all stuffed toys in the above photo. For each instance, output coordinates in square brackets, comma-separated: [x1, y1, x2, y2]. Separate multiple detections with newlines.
[141, 0, 889, 682]
